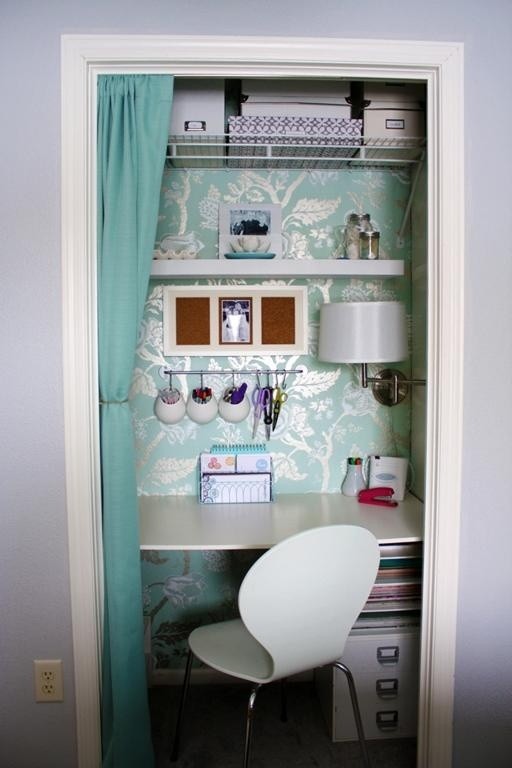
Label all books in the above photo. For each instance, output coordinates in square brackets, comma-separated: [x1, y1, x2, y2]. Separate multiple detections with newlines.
[350, 540, 425, 631]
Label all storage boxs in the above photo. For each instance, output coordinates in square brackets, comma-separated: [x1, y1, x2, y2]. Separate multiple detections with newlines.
[167, 77, 428, 168]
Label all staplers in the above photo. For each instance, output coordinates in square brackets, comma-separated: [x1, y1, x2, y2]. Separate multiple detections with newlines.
[359, 487, 398, 507]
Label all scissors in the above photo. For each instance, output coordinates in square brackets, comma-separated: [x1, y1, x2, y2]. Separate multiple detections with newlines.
[252, 387, 287, 441]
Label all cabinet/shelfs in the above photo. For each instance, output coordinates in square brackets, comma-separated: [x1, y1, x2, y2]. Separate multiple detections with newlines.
[306, 619, 422, 743]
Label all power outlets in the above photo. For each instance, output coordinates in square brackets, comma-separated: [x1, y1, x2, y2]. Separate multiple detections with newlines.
[33, 659, 63, 703]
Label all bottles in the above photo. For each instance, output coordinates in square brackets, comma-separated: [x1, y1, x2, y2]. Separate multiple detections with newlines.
[343, 213, 379, 261]
[151, 386, 186, 425]
[340, 463, 367, 497]
[186, 392, 218, 425]
[217, 386, 250, 425]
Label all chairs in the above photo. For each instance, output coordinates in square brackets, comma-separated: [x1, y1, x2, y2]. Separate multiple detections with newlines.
[169, 523, 381, 767]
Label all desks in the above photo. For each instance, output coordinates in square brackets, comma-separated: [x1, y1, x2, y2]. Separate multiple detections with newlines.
[137, 494, 424, 551]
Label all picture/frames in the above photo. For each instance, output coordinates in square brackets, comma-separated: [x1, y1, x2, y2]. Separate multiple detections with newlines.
[219, 204, 283, 262]
[162, 285, 310, 356]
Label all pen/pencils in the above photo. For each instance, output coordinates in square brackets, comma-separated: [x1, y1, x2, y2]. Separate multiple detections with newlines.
[348, 457, 363, 465]
[223, 383, 247, 404]
[157, 386, 184, 405]
[192, 387, 211, 404]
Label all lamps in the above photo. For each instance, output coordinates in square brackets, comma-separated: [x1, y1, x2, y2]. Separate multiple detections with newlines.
[318, 301, 427, 407]
